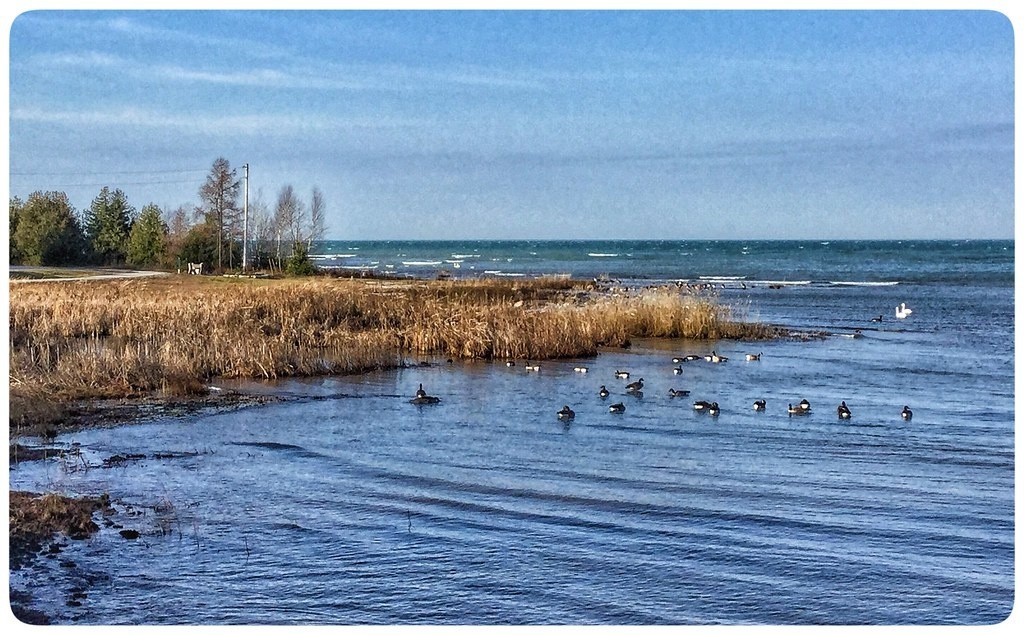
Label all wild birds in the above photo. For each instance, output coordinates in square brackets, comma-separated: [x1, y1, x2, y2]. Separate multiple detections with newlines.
[600, 385, 609, 397]
[573, 367, 580, 372]
[534, 365, 542, 371]
[871, 315, 883, 323]
[609, 402, 626, 412]
[557, 406, 575, 420]
[524, 361, 533, 371]
[673, 365, 683, 375]
[625, 377, 645, 392]
[709, 402, 720, 417]
[506, 361, 515, 367]
[668, 388, 690, 396]
[753, 400, 766, 411]
[838, 401, 852, 419]
[693, 401, 711, 411]
[615, 370, 629, 379]
[895, 306, 907, 319]
[592, 276, 746, 292]
[417, 383, 426, 398]
[581, 367, 589, 373]
[901, 303, 912, 316]
[788, 399, 811, 417]
[687, 354, 702, 361]
[711, 350, 728, 363]
[704, 355, 712, 362]
[671, 356, 685, 363]
[745, 352, 763, 362]
[901, 406, 913, 420]
